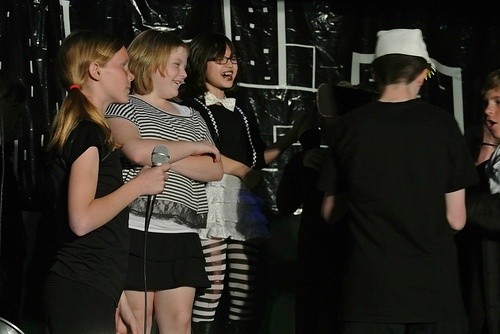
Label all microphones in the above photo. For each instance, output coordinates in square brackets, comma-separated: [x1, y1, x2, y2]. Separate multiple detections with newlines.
[145, 144, 169, 230]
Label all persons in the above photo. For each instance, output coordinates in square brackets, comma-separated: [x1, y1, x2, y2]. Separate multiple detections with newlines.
[185, 34, 264, 334]
[321, 28, 473, 334]
[104, 28, 224, 334]
[42, 29, 170, 314]
[462, 70, 500, 334]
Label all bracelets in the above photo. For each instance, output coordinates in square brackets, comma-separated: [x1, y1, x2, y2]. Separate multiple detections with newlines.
[483, 143, 498, 147]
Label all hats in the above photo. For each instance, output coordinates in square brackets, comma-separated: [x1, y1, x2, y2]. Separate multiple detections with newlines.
[371, 29, 433, 65]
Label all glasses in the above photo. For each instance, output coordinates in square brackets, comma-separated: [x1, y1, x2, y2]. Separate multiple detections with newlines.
[211, 55, 243, 64]
